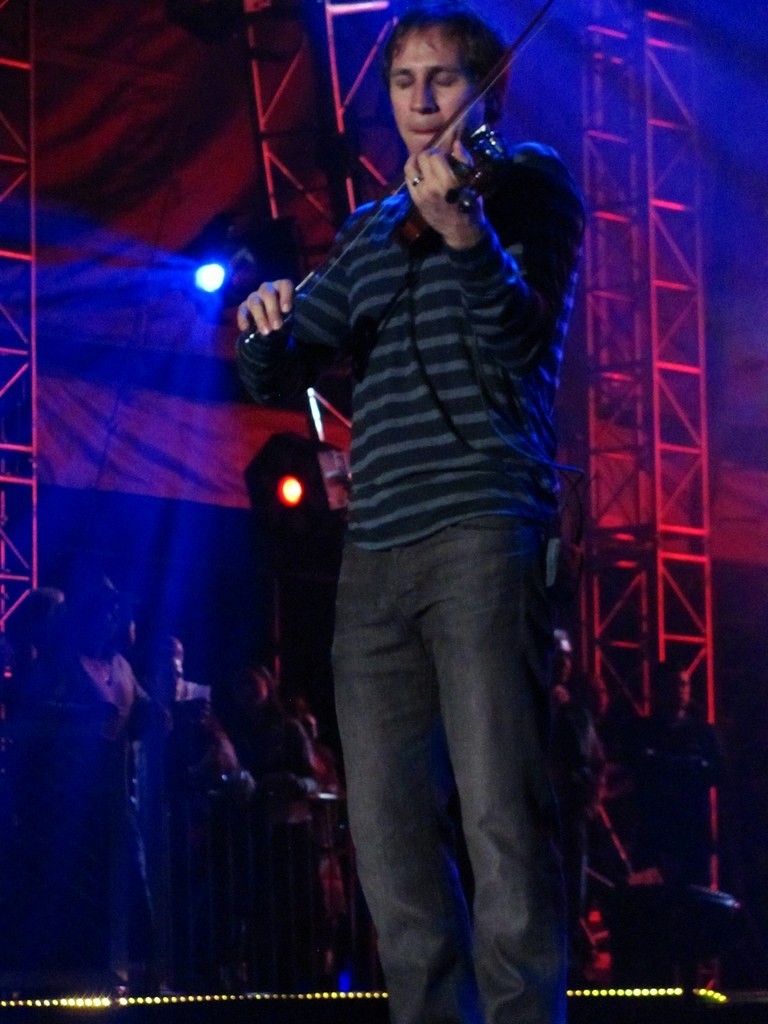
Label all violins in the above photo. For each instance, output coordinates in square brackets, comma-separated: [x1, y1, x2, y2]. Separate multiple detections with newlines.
[396, 120, 506, 245]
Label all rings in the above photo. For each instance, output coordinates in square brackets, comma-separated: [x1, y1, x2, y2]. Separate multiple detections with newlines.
[410, 175, 423, 186]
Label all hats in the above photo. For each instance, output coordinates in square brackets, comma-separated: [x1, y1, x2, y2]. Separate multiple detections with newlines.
[553, 630, 573, 653]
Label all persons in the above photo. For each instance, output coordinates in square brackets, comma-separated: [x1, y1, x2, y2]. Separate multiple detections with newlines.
[1, 629, 719, 971]
[228, 10, 583, 1024]
